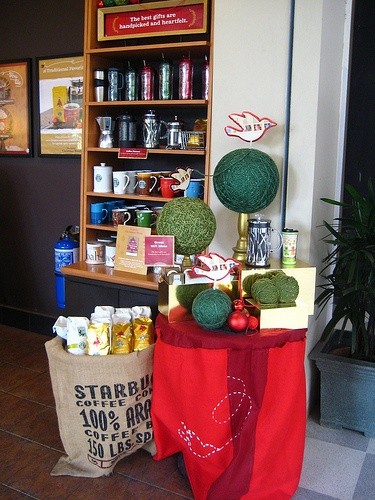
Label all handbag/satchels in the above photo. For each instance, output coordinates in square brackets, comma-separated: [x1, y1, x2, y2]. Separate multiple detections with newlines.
[44, 336, 157, 479]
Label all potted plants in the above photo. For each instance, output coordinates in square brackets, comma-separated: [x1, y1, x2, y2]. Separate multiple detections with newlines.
[307, 158, 375, 437]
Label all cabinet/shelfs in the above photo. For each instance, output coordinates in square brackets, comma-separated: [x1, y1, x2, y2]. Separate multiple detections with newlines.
[62, 0, 215, 323]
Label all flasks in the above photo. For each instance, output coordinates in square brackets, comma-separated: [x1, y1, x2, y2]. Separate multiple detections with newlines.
[93, 67, 107, 102]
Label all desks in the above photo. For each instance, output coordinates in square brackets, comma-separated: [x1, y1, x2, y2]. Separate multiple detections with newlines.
[149, 315, 307, 499]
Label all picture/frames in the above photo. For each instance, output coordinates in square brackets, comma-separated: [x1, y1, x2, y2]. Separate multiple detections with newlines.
[0, 59, 33, 157]
[32, 52, 82, 158]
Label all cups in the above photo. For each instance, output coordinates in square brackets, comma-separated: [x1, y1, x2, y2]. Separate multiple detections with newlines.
[93, 163, 112, 193]
[90, 201, 164, 228]
[85, 234, 117, 268]
[165, 115, 186, 150]
[61, 102, 82, 127]
[281, 228, 299, 265]
[183, 179, 204, 199]
[113, 170, 181, 198]
[108, 67, 124, 102]
[142, 109, 169, 149]
[117, 111, 139, 148]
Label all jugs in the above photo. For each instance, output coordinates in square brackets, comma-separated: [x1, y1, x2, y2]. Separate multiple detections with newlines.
[245, 218, 283, 268]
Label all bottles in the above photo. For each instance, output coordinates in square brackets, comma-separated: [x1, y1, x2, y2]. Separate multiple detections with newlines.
[124, 54, 140, 103]
[200, 55, 209, 100]
[178, 51, 193, 100]
[69, 78, 83, 122]
[141, 59, 154, 101]
[158, 52, 174, 100]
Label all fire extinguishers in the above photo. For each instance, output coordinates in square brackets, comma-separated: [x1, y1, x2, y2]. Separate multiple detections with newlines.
[54, 225, 79, 309]
